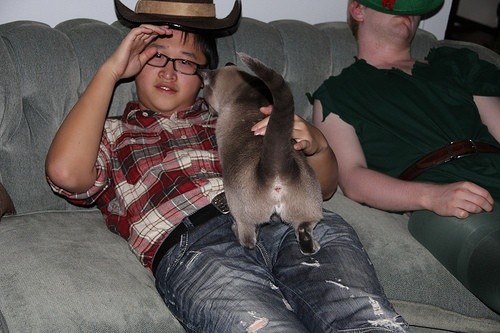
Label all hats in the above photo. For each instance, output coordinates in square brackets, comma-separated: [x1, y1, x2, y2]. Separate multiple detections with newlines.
[353, 0, 445, 16]
[113, 0, 243, 38]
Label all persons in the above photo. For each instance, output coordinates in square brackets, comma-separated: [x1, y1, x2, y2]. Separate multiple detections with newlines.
[305, 0, 500, 315]
[45, 0, 410, 333]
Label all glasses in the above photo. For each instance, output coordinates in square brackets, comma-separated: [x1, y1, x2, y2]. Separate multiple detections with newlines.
[145, 51, 210, 76]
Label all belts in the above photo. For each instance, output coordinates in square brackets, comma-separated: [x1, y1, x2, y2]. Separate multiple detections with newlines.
[151, 192, 230, 278]
[393, 136, 499, 183]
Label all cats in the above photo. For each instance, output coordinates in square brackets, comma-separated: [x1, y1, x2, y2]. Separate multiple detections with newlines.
[202, 52, 323, 256]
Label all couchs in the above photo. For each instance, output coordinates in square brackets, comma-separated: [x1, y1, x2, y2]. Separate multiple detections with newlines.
[1, 15, 499, 333]
